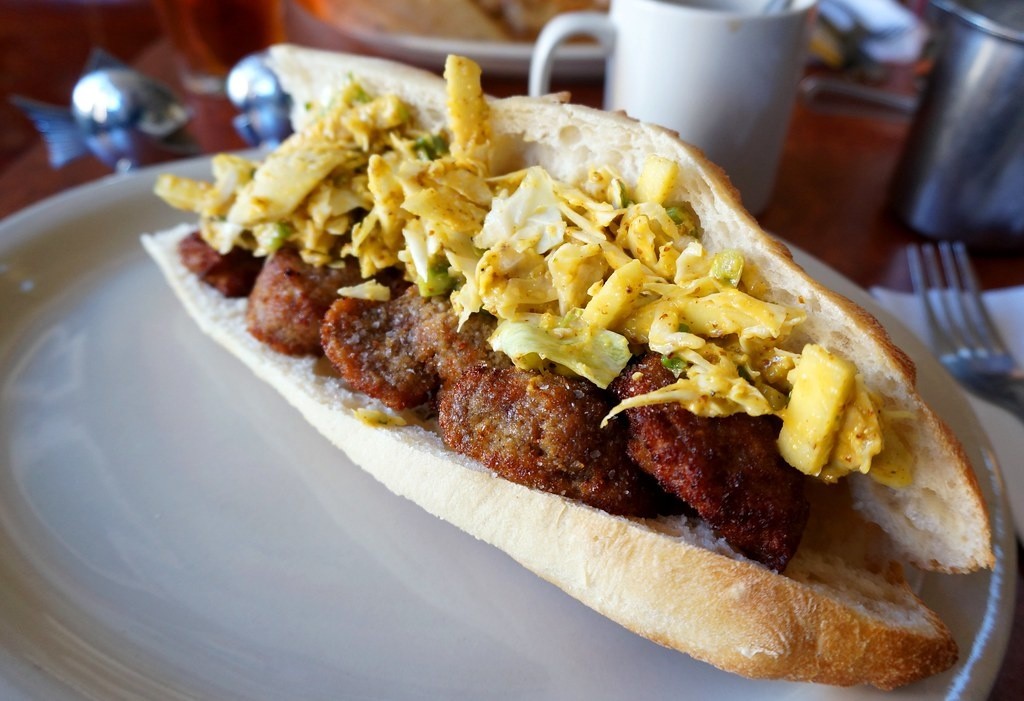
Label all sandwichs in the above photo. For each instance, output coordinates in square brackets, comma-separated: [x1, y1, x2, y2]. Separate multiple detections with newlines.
[138, 42, 994, 691]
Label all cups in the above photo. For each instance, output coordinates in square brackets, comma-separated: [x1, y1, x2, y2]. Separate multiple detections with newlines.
[529, 0, 819, 217]
[893, 0, 1024, 237]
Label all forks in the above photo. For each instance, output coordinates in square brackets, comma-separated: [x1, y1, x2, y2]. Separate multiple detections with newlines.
[908, 242, 1024, 421]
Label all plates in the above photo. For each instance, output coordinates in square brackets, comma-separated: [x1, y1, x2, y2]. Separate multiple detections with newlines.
[0, 148, 1016, 701]
[300, 0, 612, 80]
